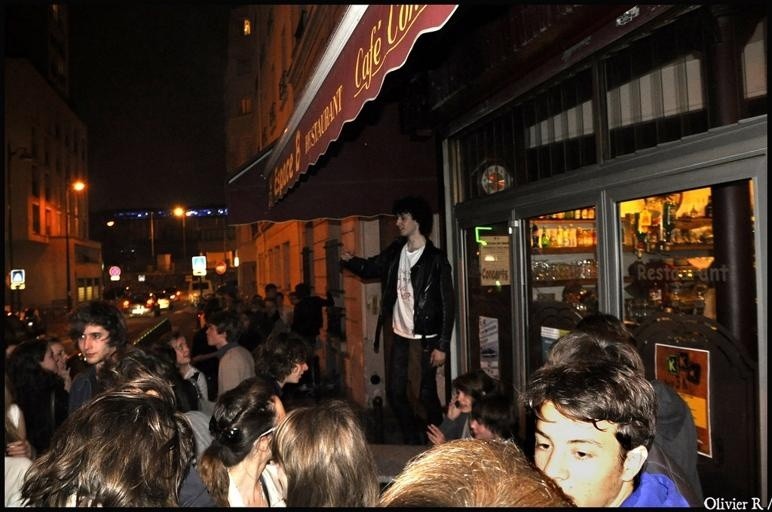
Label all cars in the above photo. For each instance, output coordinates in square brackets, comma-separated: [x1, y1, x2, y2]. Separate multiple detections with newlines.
[5, 310, 49, 365]
[122, 286, 184, 320]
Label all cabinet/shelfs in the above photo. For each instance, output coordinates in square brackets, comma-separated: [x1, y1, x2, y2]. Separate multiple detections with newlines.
[620, 213, 717, 322]
[529, 217, 598, 317]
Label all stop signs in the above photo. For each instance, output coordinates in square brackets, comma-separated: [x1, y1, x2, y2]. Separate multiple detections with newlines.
[215, 260, 228, 275]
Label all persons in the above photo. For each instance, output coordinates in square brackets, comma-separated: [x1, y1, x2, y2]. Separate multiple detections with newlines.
[336, 192, 456, 444]
[1, 279, 712, 505]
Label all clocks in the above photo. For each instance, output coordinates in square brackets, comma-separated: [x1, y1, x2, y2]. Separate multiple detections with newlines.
[477, 157, 516, 197]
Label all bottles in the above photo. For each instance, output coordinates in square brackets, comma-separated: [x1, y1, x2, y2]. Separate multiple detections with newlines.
[662, 202, 677, 243]
[704, 195, 712, 216]
[639, 206, 651, 226]
[542, 225, 595, 247]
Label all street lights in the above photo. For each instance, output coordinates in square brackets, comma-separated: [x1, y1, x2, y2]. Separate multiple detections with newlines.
[64, 181, 88, 312]
[171, 206, 188, 275]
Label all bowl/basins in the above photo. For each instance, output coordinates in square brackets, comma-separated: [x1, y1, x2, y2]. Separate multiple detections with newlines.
[686, 255, 716, 268]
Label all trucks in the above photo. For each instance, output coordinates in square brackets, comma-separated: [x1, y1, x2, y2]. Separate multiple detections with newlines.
[189, 279, 214, 307]
